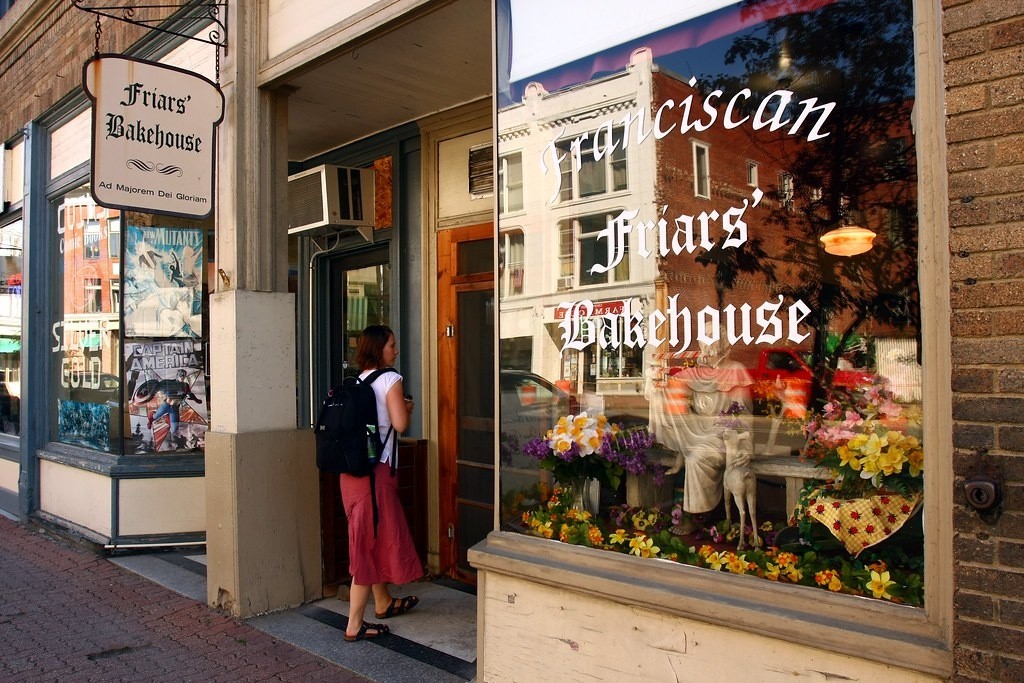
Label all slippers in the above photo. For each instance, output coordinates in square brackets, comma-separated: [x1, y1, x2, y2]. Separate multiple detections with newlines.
[344, 621, 389, 642]
[375, 596, 420, 619]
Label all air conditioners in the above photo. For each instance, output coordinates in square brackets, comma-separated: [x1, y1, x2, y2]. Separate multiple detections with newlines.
[288, 164, 376, 236]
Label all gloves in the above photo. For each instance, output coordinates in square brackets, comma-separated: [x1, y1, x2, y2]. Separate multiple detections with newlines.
[188, 394, 202, 404]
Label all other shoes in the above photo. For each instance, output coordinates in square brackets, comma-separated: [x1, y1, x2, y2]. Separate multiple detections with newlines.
[147, 411, 157, 428]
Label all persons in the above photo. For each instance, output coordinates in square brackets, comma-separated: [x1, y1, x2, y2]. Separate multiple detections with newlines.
[643, 320, 756, 536]
[312, 324, 424, 642]
[147, 369, 203, 434]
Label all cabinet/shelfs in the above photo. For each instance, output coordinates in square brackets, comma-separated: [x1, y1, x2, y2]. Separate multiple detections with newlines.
[346, 329, 361, 371]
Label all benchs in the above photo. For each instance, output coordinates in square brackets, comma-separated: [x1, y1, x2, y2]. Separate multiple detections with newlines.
[623, 455, 844, 522]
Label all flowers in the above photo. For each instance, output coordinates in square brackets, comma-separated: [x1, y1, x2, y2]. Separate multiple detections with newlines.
[520, 358, 923, 609]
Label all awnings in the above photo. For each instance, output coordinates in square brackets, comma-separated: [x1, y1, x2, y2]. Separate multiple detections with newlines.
[0, 338, 21, 353]
[77, 334, 100, 347]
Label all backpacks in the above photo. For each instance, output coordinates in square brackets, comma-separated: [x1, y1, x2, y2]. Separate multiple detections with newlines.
[314, 368, 402, 477]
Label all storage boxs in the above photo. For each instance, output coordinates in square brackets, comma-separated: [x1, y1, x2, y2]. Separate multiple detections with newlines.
[347, 296, 369, 331]
[347, 284, 365, 297]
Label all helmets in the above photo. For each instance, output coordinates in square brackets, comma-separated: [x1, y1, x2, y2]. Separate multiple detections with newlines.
[177, 369, 187, 376]
